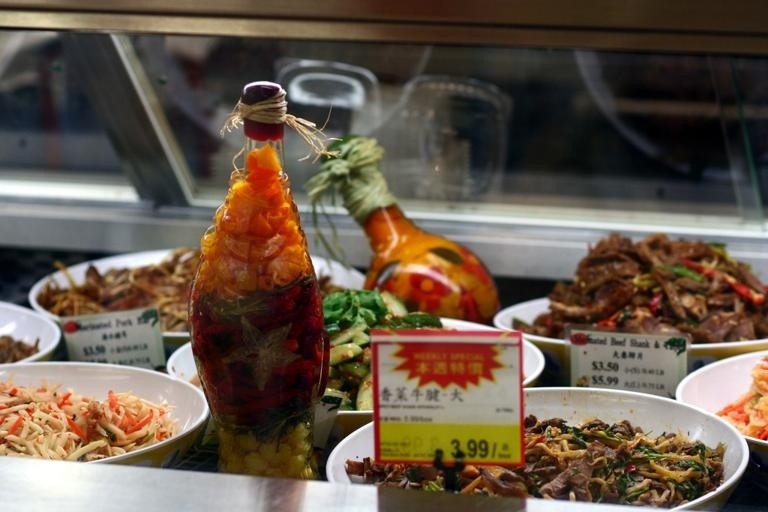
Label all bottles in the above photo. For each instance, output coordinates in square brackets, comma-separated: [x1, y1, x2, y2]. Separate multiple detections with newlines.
[188, 79, 331, 480]
[319, 134, 499, 323]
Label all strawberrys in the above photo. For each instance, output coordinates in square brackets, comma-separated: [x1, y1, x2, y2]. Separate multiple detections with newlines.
[185, 273, 329, 430]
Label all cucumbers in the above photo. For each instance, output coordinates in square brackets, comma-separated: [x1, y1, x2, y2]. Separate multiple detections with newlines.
[324, 284, 443, 413]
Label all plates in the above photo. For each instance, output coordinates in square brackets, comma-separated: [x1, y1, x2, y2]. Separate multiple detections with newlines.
[165, 314, 547, 459]
[26, 248, 371, 356]
[2, 363, 213, 475]
[673, 348, 767, 471]
[326, 387, 753, 512]
[0, 300, 66, 362]
[489, 289, 767, 386]
